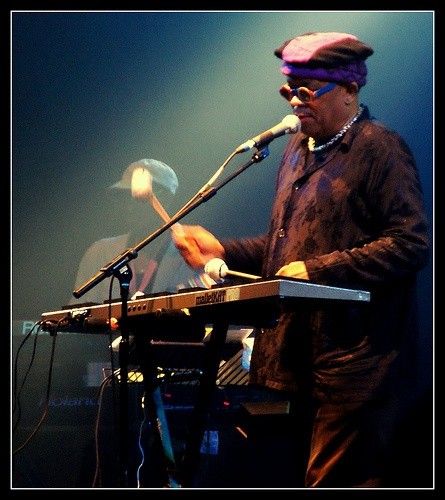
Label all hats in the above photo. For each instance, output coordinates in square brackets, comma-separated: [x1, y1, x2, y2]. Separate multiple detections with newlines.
[109, 158, 179, 198]
[281, 30, 374, 86]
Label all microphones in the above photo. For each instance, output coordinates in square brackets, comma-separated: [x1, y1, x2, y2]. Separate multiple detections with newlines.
[235, 115, 301, 155]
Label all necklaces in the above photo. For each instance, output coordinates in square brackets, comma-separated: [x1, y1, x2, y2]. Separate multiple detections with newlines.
[306, 108, 364, 155]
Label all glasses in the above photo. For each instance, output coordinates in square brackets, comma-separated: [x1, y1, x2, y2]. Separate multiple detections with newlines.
[279, 81, 337, 101]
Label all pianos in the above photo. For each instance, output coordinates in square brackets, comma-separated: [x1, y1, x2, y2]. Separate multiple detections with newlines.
[41, 276, 371, 327]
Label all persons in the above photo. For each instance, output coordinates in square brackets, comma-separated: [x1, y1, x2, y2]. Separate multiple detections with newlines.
[167, 31, 430, 489]
[65, 157, 223, 378]
